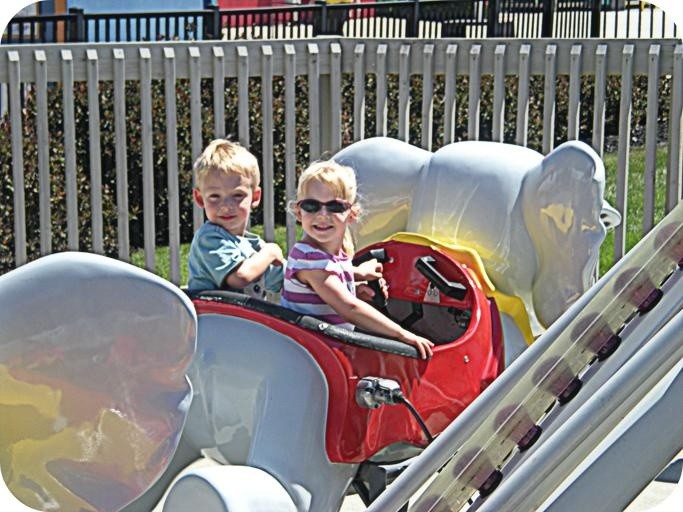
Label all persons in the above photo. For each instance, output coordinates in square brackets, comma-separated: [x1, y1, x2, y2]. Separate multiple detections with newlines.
[186, 138, 284, 303]
[279, 160, 434, 360]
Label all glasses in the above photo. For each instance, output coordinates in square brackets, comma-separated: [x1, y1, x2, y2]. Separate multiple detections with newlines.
[297, 199, 352, 213]
